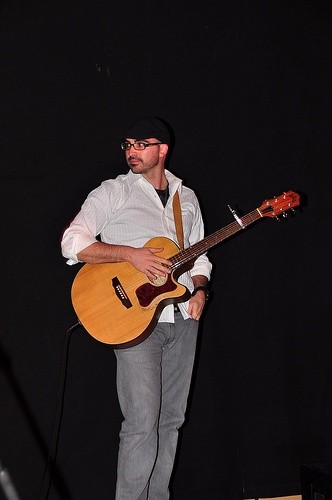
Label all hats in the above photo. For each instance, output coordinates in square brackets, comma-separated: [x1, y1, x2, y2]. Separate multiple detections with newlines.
[121, 115, 170, 147]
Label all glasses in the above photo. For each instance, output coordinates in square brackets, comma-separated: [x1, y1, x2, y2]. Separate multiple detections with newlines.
[120, 143, 165, 150]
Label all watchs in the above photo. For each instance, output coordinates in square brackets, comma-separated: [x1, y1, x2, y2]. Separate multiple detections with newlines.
[192, 286, 210, 301]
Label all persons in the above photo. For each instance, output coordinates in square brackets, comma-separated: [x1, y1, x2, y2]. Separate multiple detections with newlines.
[61, 115, 213, 500]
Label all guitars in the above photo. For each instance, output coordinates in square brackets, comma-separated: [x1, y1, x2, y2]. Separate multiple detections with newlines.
[68, 189, 301, 350]
